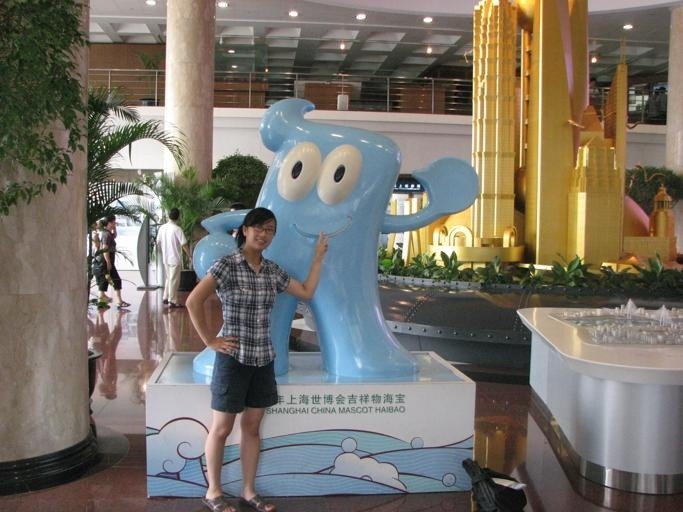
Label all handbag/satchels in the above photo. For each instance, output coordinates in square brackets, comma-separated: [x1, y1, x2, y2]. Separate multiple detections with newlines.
[462, 458, 528, 512]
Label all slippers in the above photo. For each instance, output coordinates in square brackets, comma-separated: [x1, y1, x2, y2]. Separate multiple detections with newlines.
[202, 496, 237, 512]
[238, 494, 277, 512]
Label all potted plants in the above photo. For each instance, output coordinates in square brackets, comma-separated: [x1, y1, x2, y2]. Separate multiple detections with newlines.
[132, 165, 234, 294]
[133, 49, 165, 106]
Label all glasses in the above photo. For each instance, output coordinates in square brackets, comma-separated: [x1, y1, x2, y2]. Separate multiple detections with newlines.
[254, 224, 275, 234]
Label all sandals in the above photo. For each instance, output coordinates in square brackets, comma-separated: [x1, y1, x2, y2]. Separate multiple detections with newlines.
[117, 302, 130, 307]
[164, 300, 184, 308]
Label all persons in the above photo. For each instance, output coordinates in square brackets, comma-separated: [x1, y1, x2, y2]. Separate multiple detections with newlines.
[183, 207, 330, 512]
[84, 305, 107, 393]
[91, 214, 130, 308]
[587, 76, 603, 122]
[85, 219, 112, 303]
[160, 308, 180, 357]
[643, 85, 667, 125]
[155, 207, 190, 308]
[99, 308, 131, 399]
